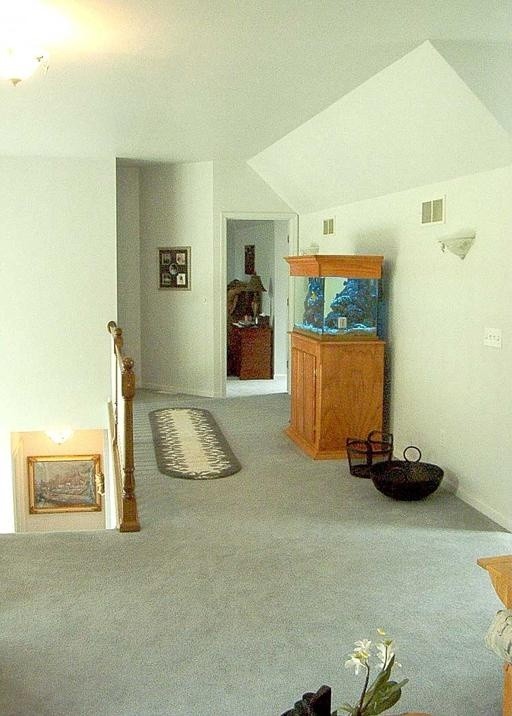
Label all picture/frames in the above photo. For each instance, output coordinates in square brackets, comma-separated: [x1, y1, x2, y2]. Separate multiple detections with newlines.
[243, 242, 255, 275]
[156, 245, 192, 292]
[27, 454, 102, 514]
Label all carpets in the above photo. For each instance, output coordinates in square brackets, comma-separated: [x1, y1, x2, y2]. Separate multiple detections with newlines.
[148, 407, 242, 481]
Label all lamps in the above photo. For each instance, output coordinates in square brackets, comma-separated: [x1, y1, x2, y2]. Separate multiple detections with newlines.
[299, 246, 319, 256]
[243, 274, 267, 320]
[437, 235, 476, 259]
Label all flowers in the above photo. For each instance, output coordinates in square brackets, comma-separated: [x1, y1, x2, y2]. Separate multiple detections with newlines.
[344, 627, 410, 715]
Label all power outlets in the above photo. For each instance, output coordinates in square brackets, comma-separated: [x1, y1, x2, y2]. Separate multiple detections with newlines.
[482, 326, 503, 349]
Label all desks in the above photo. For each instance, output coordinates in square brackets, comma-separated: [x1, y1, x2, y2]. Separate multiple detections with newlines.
[477, 554, 512, 715]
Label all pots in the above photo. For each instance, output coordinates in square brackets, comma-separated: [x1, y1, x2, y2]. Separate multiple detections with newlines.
[370, 446, 443, 501]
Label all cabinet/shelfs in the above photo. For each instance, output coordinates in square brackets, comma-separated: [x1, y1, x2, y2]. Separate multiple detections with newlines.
[232, 325, 272, 380]
[283, 331, 386, 461]
